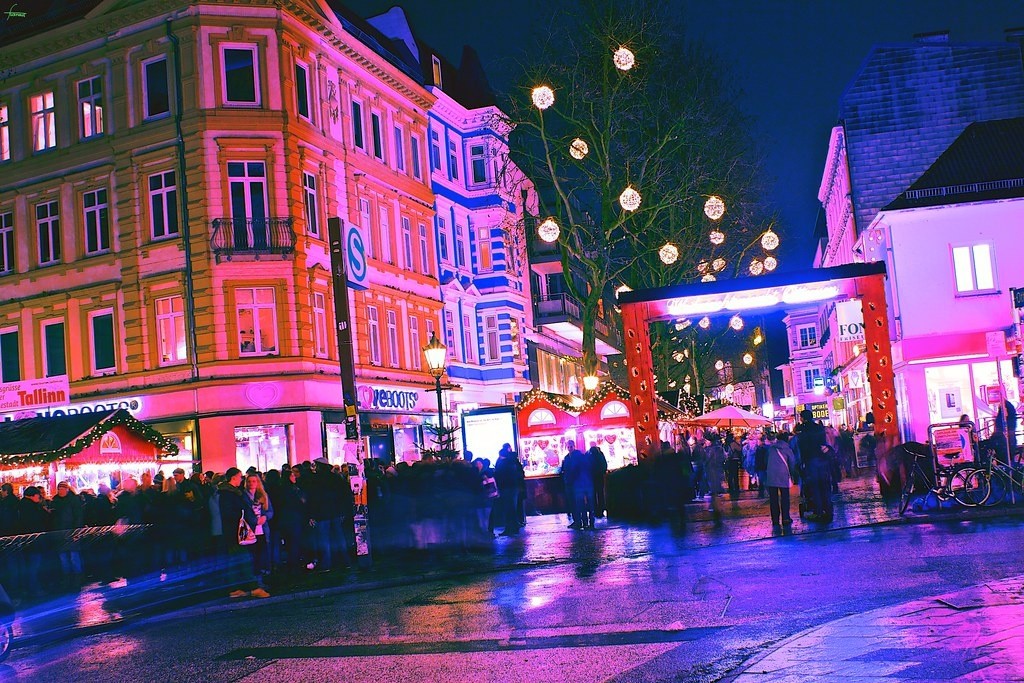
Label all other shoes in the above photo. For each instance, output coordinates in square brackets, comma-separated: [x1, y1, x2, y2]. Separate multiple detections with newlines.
[594, 513, 605, 518]
[772, 518, 793, 526]
[307, 562, 317, 569]
[567, 522, 595, 529]
[809, 514, 834, 522]
[498, 521, 526, 536]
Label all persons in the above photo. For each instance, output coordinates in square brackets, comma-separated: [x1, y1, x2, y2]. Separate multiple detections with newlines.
[557, 440, 607, 529]
[657, 409, 855, 534]
[959, 400, 1016, 467]
[0, 442, 526, 602]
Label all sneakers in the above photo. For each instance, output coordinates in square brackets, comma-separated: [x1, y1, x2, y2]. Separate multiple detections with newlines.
[229, 590, 247, 598]
[251, 588, 270, 598]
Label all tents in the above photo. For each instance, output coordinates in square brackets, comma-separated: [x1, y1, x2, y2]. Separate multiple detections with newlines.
[1, 408, 180, 500]
[515, 380, 689, 477]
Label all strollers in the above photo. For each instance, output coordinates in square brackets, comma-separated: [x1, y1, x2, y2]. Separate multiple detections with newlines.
[797, 460, 834, 520]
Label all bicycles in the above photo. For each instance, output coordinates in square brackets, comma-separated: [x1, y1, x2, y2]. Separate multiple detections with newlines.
[965, 438, 1024, 508]
[898, 443, 992, 515]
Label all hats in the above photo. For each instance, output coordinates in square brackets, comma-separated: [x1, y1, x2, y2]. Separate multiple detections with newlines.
[58, 481, 69, 489]
[98, 484, 111, 495]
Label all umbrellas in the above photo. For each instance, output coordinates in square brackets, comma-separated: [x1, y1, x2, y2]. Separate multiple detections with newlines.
[676, 405, 774, 432]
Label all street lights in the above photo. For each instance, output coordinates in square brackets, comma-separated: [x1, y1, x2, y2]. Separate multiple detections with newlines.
[423, 329, 453, 459]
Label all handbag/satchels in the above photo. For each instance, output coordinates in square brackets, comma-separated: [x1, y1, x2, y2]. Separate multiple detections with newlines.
[238, 510, 257, 545]
[789, 475, 794, 488]
